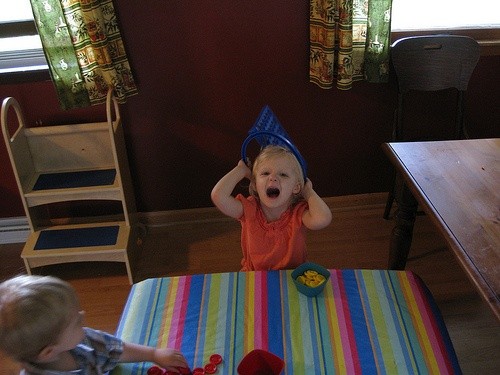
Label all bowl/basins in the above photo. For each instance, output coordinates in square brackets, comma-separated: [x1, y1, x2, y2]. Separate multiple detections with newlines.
[237, 349, 285, 375]
[291, 261, 330, 297]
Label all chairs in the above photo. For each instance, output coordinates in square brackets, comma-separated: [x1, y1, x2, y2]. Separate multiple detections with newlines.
[383, 35, 480, 219]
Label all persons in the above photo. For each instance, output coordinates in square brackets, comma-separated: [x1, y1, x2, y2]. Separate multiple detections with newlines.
[0, 275, 188, 375]
[211, 144, 333, 272]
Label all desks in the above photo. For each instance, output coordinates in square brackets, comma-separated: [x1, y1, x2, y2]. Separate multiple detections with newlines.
[381, 138, 500, 326]
